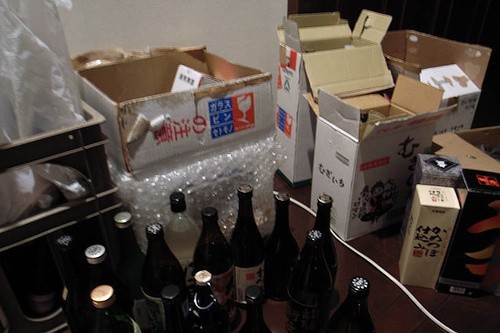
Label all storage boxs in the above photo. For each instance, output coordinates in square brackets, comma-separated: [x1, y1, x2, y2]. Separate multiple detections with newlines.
[301, 74, 458, 241]
[276, 8, 396, 186]
[70, 45, 277, 174]
[382, 29, 492, 135]
[398, 127, 500, 297]
[1, 97, 125, 285]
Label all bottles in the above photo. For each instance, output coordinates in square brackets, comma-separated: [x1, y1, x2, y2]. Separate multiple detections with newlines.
[56, 236, 90, 332]
[88, 283, 142, 333]
[184, 270, 229, 333]
[81, 243, 135, 333]
[234, 285, 273, 333]
[285, 230, 333, 333]
[263, 191, 300, 302]
[324, 276, 376, 333]
[230, 183, 265, 311]
[139, 222, 190, 333]
[191, 206, 237, 322]
[160, 284, 189, 333]
[107, 210, 155, 333]
[310, 194, 338, 282]
[163, 191, 201, 305]
